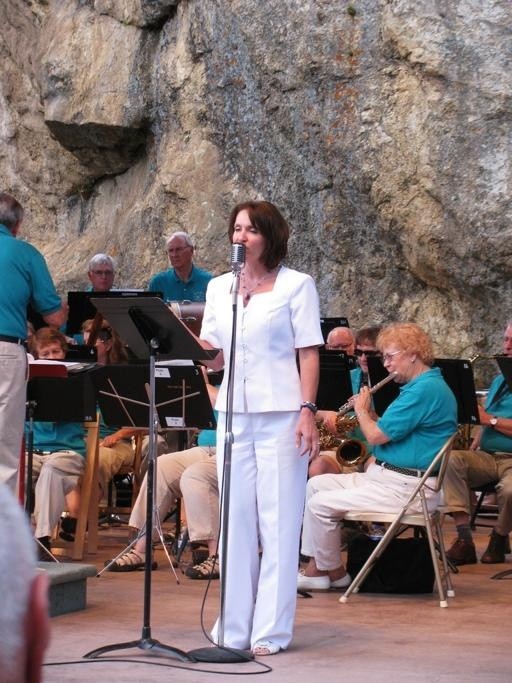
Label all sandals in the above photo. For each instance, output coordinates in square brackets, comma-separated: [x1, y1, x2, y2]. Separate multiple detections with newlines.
[105, 548, 158, 571]
[185, 555, 220, 579]
[252, 641, 279, 656]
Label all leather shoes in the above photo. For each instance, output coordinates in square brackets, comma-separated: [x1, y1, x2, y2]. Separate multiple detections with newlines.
[440, 536, 478, 563]
[482, 535, 506, 563]
[58, 514, 88, 543]
[296, 570, 351, 592]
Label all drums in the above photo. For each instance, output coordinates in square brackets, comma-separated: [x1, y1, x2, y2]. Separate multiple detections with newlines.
[170, 299, 204, 335]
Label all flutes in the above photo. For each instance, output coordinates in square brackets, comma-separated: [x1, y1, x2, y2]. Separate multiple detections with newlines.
[336, 368, 399, 415]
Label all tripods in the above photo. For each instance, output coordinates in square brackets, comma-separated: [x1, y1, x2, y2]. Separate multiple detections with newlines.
[96, 420, 185, 585]
[392, 524, 459, 574]
[82, 356, 196, 660]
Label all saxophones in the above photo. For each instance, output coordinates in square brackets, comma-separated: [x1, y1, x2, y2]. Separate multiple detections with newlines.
[319, 412, 372, 466]
[456, 354, 507, 450]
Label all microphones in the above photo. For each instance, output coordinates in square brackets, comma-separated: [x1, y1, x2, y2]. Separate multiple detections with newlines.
[231, 243, 245, 274]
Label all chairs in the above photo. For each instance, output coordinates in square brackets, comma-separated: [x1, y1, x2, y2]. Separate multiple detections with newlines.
[50, 411, 193, 572]
[338, 424, 466, 611]
[470, 480, 502, 531]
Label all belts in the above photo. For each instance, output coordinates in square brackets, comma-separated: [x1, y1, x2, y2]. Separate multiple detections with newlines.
[376, 460, 426, 478]
[0, 336, 27, 348]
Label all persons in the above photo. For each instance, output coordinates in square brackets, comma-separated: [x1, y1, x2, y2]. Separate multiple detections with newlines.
[1, 480, 52, 683]
[296, 320, 512, 591]
[2, 190, 221, 579]
[179, 199, 324, 658]
